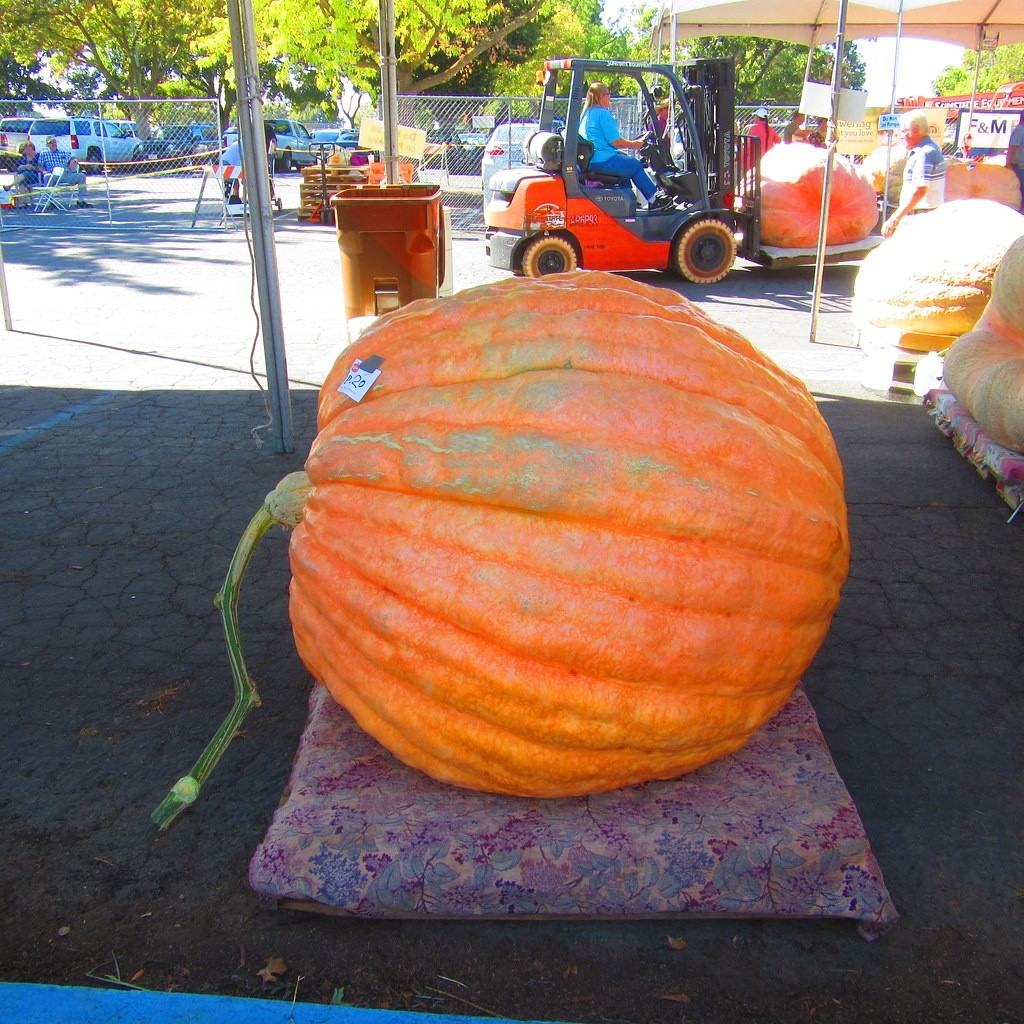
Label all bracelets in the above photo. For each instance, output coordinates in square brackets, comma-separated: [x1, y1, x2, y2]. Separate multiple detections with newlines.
[891, 214, 901, 222]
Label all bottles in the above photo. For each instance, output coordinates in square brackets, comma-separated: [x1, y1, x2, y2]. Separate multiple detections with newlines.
[914, 351, 944, 397]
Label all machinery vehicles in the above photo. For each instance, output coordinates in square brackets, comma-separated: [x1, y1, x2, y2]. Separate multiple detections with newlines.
[484, 56, 889, 289]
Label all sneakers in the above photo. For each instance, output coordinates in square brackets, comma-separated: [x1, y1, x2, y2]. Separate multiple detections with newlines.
[648, 195, 672, 211]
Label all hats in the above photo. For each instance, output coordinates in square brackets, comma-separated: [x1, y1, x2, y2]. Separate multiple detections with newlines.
[752, 108, 767, 118]
[45, 135, 55, 144]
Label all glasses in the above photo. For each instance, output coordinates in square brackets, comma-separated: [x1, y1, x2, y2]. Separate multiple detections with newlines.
[602, 90, 610, 95]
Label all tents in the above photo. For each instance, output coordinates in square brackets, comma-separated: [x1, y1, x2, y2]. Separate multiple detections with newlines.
[649, 0, 1024, 343]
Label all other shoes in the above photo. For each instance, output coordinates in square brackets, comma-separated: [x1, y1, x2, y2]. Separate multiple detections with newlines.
[47, 204, 53, 210]
[21, 202, 27, 209]
[77, 201, 92, 208]
[3, 185, 9, 191]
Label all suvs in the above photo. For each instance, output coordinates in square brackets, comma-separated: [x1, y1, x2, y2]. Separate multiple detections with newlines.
[26, 114, 147, 173]
[130, 124, 228, 168]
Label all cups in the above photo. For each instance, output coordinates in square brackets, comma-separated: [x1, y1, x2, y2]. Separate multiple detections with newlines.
[317, 156, 321, 165]
[368, 155, 374, 166]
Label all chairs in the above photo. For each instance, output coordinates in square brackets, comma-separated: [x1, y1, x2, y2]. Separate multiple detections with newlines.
[58, 164, 84, 208]
[561, 128, 620, 188]
[32, 167, 68, 213]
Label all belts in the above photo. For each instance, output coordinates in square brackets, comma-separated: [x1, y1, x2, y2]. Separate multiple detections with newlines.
[907, 208, 935, 215]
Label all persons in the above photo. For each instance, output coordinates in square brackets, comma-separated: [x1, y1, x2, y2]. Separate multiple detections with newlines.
[218, 141, 241, 199]
[739, 108, 782, 178]
[1005, 110, 1024, 211]
[578, 82, 674, 213]
[784, 108, 828, 144]
[880, 108, 946, 239]
[3, 141, 41, 209]
[263, 123, 277, 155]
[37, 135, 93, 209]
[645, 101, 671, 153]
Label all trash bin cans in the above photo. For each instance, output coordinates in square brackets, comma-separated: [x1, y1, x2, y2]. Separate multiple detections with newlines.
[329, 182, 446, 345]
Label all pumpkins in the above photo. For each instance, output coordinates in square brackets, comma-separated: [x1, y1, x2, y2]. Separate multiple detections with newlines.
[152, 273, 850, 834]
[736, 141, 879, 247]
[859, 146, 910, 203]
[945, 163, 1022, 211]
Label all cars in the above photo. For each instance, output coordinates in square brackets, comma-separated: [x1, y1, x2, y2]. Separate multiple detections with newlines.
[424, 130, 485, 174]
[308, 129, 345, 148]
[0, 116, 38, 172]
[328, 129, 378, 168]
[220, 118, 323, 173]
[483, 122, 544, 194]
